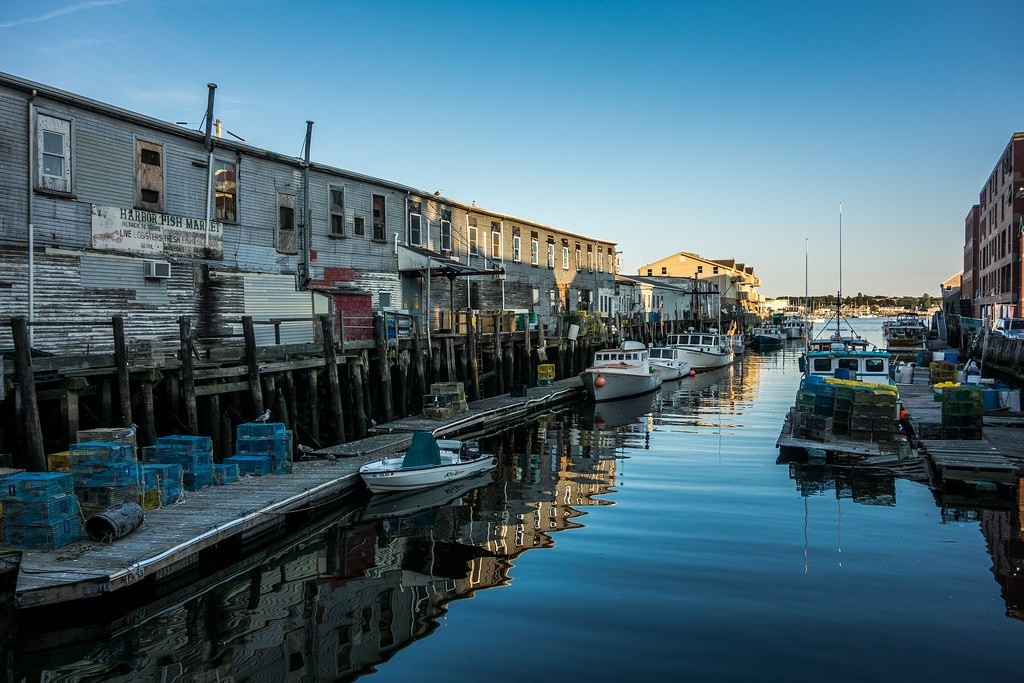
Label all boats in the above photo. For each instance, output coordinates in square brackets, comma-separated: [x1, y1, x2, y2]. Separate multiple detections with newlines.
[775, 202, 912, 458]
[646, 340, 691, 381]
[358, 431, 499, 493]
[578, 340, 663, 404]
[751, 314, 808, 346]
[666, 293, 746, 369]
[882, 311, 925, 347]
[358, 467, 498, 526]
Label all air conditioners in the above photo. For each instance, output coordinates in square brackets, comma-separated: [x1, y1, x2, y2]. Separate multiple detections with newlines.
[143, 262, 171, 278]
[495, 264, 506, 280]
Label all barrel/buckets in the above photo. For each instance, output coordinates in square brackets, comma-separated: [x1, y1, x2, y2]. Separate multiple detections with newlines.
[945, 352, 958, 364]
[84, 497, 144, 539]
[981, 390, 1000, 412]
[834, 368, 849, 380]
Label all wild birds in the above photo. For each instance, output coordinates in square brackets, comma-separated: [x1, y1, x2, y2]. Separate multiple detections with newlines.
[434, 189, 442, 196]
[472, 199, 477, 207]
